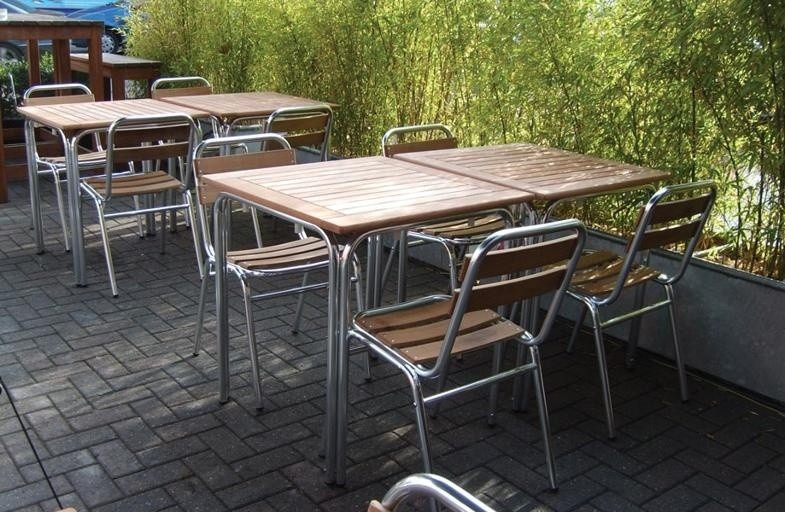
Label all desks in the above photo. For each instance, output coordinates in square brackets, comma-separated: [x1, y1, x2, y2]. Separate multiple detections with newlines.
[195, 154, 539, 487]
[16, 97, 212, 291]
[160, 88, 341, 168]
[391, 142, 676, 412]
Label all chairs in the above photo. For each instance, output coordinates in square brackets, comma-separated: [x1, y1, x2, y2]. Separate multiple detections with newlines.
[364, 473, 496, 511]
[334, 218, 586, 490]
[550, 181, 716, 444]
[150, 77, 248, 235]
[247, 103, 333, 248]
[381, 124, 535, 305]
[191, 133, 374, 411]
[21, 83, 144, 251]
[80, 115, 205, 298]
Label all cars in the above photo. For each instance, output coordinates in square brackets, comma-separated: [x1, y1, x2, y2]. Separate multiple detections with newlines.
[0, 0, 148, 61]
